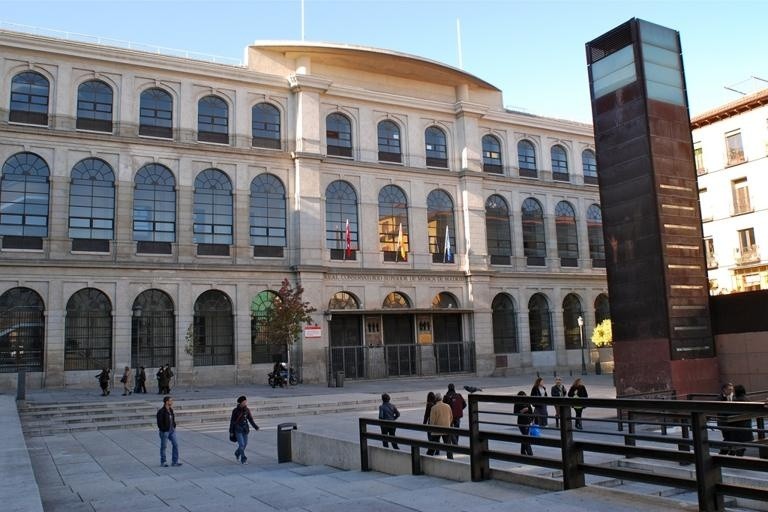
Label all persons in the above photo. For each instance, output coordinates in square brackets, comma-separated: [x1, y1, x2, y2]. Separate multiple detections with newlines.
[94, 364, 174, 395]
[423, 384, 467, 459]
[157, 396, 183, 467]
[514, 378, 588, 457]
[379, 394, 400, 450]
[229, 396, 259, 465]
[272, 360, 287, 388]
[716, 383, 735, 454]
[725, 385, 754, 457]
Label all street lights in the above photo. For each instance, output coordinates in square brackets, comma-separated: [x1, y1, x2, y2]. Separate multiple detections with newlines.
[577, 314, 587, 377]
[133, 303, 143, 382]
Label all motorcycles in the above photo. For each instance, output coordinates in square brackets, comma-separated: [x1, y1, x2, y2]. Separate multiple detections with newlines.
[267, 364, 298, 388]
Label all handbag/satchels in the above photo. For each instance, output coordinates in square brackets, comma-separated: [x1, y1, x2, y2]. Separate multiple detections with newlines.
[529, 424, 539, 436]
[230, 433, 237, 442]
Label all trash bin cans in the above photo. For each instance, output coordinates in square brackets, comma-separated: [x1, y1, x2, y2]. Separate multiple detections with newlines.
[336, 371, 344, 387]
[277, 423, 297, 463]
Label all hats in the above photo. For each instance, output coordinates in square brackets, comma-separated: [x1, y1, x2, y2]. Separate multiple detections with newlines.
[237, 396, 246, 402]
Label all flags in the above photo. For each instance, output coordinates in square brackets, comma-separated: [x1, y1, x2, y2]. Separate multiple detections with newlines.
[446, 228, 451, 260]
[347, 222, 350, 256]
[399, 225, 406, 260]
